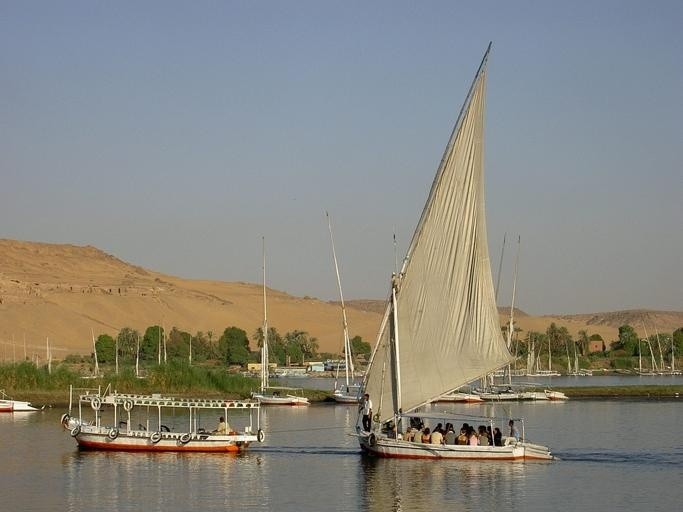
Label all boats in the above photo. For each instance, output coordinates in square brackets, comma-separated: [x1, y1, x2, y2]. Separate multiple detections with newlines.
[60, 382, 269, 454]
[0, 389, 41, 412]
[70, 445, 250, 465]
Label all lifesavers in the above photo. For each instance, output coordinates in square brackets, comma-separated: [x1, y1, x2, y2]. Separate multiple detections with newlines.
[367, 433, 378, 447]
[180, 433, 191, 443]
[123, 399, 134, 411]
[139, 423, 147, 430]
[91, 398, 102, 411]
[119, 421, 126, 424]
[61, 414, 69, 425]
[258, 429, 264, 443]
[108, 427, 119, 439]
[159, 425, 170, 432]
[150, 432, 162, 443]
[71, 425, 80, 437]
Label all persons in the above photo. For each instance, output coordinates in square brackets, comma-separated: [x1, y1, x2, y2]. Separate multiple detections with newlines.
[211, 416, 233, 435]
[360, 393, 373, 433]
[403, 423, 502, 447]
[508, 419, 519, 442]
[390, 424, 395, 437]
